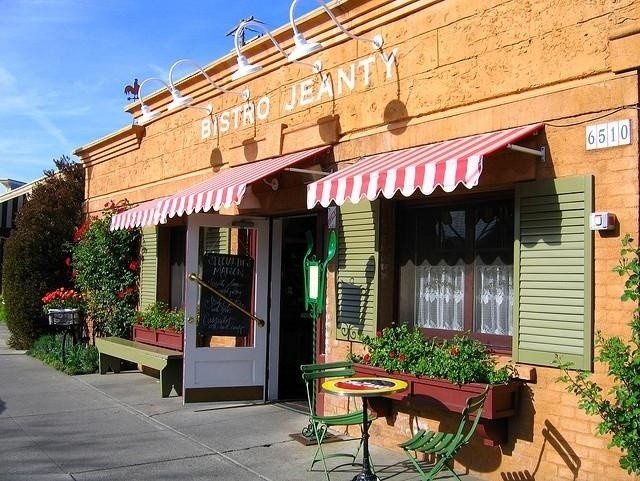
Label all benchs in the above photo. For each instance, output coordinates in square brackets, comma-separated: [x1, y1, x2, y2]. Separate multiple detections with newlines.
[96, 336, 184, 399]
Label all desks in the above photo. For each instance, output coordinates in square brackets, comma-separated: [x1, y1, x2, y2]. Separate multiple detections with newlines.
[320, 377, 409, 481]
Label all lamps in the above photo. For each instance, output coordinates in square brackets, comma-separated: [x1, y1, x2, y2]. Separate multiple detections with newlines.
[168, 59, 249, 113]
[137, 77, 213, 125]
[232, 21, 322, 82]
[285, 0, 385, 63]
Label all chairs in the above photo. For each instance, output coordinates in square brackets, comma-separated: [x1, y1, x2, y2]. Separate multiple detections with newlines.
[299, 360, 379, 481]
[498, 418, 581, 481]
[397, 385, 490, 481]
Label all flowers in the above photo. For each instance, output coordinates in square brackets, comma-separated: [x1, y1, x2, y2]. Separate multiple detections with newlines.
[408, 328, 513, 385]
[345, 318, 430, 377]
[40, 288, 84, 310]
[127, 302, 187, 334]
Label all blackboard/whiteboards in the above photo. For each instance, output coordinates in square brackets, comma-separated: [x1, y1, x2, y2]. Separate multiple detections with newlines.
[200, 252, 254, 337]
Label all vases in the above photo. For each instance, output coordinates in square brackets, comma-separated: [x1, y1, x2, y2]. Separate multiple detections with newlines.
[353, 358, 412, 401]
[413, 374, 522, 420]
[48, 308, 90, 367]
[132, 323, 184, 352]
